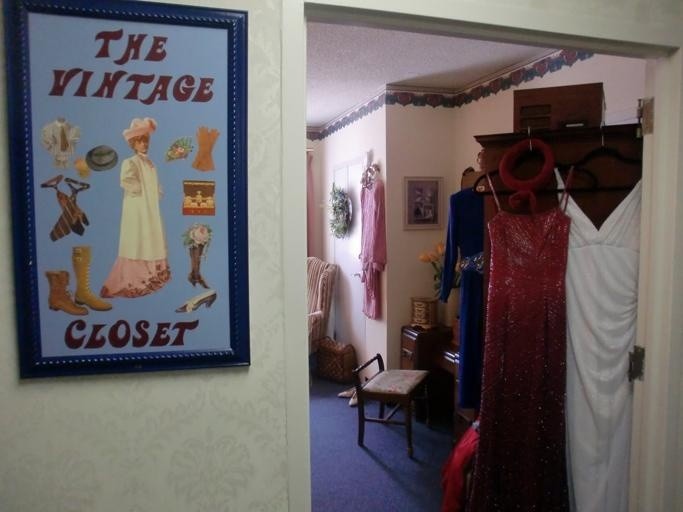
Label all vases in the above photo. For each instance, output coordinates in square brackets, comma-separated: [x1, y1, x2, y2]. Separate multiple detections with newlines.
[438, 286, 460, 326]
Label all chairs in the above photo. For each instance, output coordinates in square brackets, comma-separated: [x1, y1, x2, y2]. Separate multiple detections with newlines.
[307, 256, 337, 355]
[351, 353, 429, 458]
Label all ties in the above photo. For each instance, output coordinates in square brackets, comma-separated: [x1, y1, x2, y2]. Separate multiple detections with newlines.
[59, 126, 70, 152]
[38, 173, 91, 242]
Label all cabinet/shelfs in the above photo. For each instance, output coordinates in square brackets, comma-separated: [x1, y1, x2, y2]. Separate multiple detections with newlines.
[400, 325, 478, 442]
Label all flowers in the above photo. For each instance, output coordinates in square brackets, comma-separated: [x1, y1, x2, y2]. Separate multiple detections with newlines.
[417, 240, 461, 296]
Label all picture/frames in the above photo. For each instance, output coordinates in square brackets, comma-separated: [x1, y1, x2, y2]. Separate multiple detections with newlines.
[403, 175, 444, 230]
[4, 0, 250, 379]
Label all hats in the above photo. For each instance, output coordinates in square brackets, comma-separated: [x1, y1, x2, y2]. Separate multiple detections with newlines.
[84, 143, 120, 172]
[120, 115, 158, 141]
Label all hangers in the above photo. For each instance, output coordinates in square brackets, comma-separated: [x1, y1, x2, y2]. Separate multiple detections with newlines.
[562, 119, 643, 194]
[473, 124, 598, 195]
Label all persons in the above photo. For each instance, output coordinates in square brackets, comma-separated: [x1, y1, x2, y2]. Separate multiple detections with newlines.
[100, 115, 171, 297]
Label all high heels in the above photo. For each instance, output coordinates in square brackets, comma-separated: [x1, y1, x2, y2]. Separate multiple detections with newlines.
[174, 290, 218, 313]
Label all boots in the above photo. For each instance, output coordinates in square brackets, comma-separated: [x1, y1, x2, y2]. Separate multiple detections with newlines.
[70, 245, 114, 312]
[186, 241, 213, 289]
[43, 268, 90, 316]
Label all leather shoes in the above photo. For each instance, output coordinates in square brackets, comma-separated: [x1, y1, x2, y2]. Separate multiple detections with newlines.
[335, 382, 369, 408]
